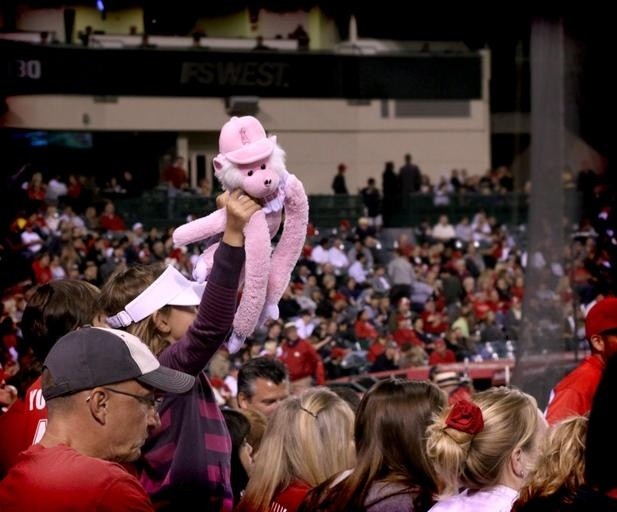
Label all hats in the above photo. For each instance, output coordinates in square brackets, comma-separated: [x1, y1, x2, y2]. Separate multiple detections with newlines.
[41, 323, 196, 401]
[106, 264, 208, 328]
[585, 296, 617, 339]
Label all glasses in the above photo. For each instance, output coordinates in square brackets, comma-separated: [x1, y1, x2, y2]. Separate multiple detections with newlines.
[86, 387, 168, 415]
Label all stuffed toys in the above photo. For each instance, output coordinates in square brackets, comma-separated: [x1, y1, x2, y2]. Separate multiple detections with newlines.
[173, 116, 309, 354]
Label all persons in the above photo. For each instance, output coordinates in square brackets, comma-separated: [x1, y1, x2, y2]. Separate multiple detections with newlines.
[1, 153, 617, 512]
[39, 23, 308, 53]
